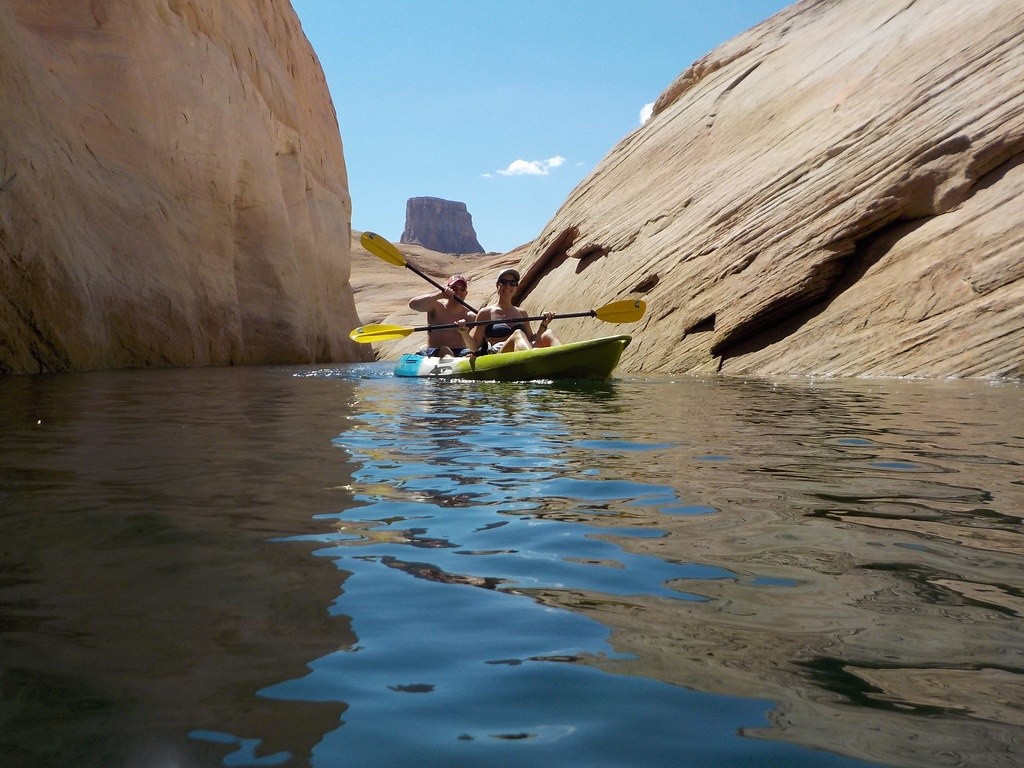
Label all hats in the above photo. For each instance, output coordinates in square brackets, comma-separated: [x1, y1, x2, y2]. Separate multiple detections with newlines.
[447, 275, 467, 288]
[494, 268, 521, 290]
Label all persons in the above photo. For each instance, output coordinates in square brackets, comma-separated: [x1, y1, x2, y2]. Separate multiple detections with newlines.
[454, 267, 562, 355]
[409, 275, 476, 358]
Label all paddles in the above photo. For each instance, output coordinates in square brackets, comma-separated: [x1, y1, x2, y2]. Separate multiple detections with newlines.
[359, 229, 480, 316]
[348, 298, 648, 345]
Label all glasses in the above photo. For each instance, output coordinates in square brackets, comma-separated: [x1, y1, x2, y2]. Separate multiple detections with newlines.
[498, 278, 518, 286]
[452, 286, 466, 291]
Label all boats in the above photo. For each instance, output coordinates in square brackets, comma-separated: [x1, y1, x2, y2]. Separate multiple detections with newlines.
[391, 332, 637, 379]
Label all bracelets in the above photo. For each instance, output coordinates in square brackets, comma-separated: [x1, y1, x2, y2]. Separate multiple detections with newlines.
[541, 323, 547, 329]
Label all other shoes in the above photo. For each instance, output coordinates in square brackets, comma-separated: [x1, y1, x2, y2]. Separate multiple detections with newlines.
[440, 345, 455, 358]
